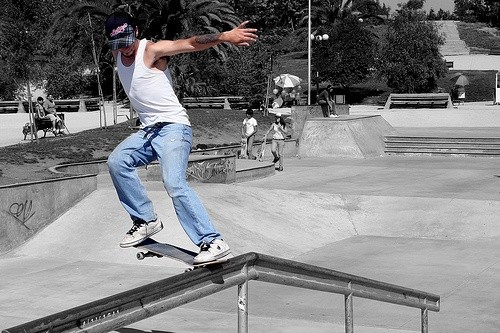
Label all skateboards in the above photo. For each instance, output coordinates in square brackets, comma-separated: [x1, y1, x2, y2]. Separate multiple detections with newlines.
[241, 135, 247, 159]
[257, 134, 265, 162]
[133, 238, 227, 272]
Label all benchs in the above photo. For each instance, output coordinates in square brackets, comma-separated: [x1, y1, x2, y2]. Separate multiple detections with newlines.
[23, 114, 64, 139]
[263, 98, 298, 108]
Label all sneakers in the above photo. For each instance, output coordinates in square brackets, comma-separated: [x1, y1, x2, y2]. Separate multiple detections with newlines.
[335, 114, 340, 118]
[120, 217, 164, 247]
[193, 239, 231, 262]
[330, 115, 337, 118]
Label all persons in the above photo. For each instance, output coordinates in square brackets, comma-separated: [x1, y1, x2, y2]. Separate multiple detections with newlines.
[34, 95, 57, 134]
[317, 83, 341, 117]
[43, 93, 66, 134]
[105, 9, 259, 268]
[241, 108, 258, 160]
[273, 87, 303, 107]
[264, 112, 288, 171]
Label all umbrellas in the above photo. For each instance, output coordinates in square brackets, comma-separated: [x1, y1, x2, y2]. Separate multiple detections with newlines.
[272, 73, 303, 88]
[450, 74, 469, 86]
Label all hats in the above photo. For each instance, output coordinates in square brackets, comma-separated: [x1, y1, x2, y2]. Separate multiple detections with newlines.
[275, 113, 281, 117]
[105, 11, 135, 50]
